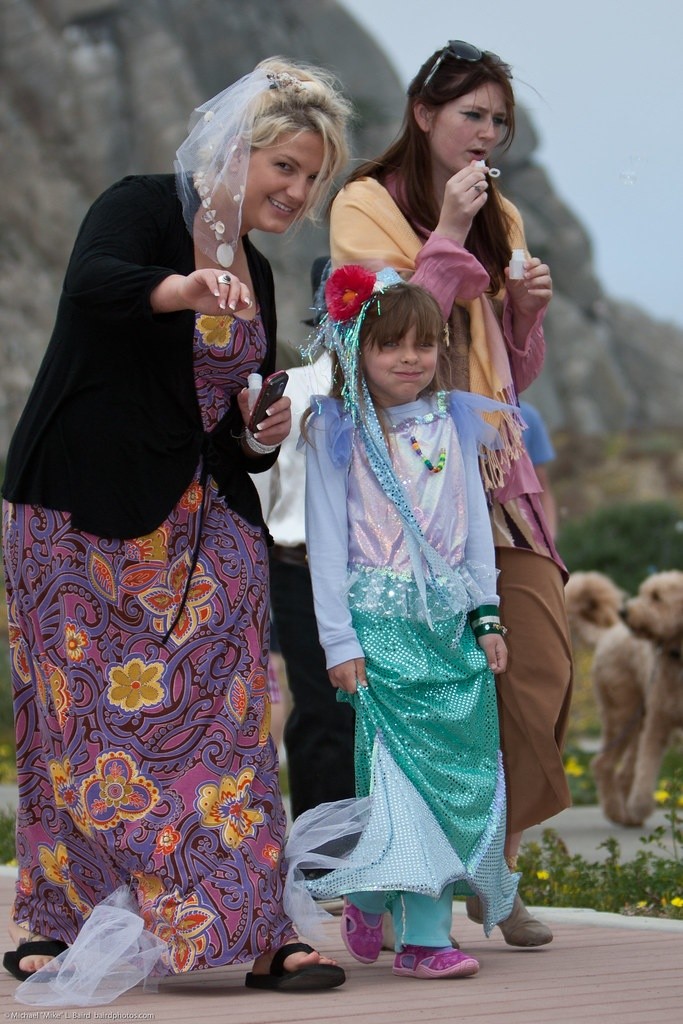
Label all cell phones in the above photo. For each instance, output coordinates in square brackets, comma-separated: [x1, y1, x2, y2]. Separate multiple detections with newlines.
[247, 370, 289, 434]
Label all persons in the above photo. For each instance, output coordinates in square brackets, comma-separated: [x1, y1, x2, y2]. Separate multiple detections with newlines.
[240, 253, 367, 917]
[324, 42, 576, 947]
[0, 57, 346, 994]
[303, 282, 512, 981]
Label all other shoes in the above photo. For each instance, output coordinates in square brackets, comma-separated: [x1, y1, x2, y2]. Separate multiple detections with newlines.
[340, 892, 384, 964]
[292, 868, 345, 911]
[379, 913, 461, 952]
[466, 889, 553, 946]
[393, 944, 480, 978]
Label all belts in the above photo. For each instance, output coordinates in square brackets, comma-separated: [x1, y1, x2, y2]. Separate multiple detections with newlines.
[270, 543, 311, 569]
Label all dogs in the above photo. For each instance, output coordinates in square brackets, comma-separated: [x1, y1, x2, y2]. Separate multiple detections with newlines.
[563, 569, 683, 827]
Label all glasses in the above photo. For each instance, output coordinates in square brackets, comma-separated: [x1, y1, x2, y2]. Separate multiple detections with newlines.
[422, 38, 513, 89]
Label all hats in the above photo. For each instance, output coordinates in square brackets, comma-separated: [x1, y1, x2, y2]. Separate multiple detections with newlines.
[301, 258, 332, 329]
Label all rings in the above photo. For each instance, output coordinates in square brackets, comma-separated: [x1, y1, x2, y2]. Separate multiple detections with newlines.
[217, 274, 231, 285]
[472, 185, 482, 194]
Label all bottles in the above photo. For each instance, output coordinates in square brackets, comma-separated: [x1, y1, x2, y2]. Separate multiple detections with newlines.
[509, 249, 527, 279]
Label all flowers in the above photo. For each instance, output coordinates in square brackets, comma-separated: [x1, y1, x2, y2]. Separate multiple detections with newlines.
[323, 264, 376, 324]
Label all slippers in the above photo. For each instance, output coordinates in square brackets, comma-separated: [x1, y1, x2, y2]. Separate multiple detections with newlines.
[245, 943, 346, 990]
[3, 939, 74, 982]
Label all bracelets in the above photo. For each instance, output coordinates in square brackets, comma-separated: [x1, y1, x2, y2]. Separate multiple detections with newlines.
[470, 615, 501, 630]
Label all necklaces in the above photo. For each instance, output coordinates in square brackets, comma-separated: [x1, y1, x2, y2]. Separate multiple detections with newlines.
[369, 385, 448, 472]
